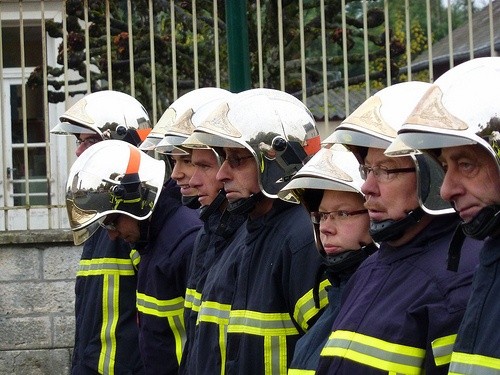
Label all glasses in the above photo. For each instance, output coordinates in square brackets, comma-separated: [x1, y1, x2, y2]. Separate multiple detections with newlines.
[310, 207, 369, 225]
[220, 153, 255, 170]
[74, 137, 99, 146]
[356, 162, 416, 181]
[99, 213, 122, 232]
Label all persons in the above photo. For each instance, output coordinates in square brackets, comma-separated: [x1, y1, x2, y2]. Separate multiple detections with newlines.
[384, 56, 500, 375]
[278, 141, 380, 375]
[48, 86, 321, 375]
[315, 81, 490, 375]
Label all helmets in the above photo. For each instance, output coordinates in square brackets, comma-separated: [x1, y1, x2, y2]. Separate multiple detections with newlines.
[383, 58, 500, 184]
[182, 87, 320, 199]
[277, 144, 379, 259]
[157, 93, 237, 167]
[137, 86, 231, 157]
[50, 89, 153, 148]
[321, 81, 459, 216]
[66, 139, 166, 245]
